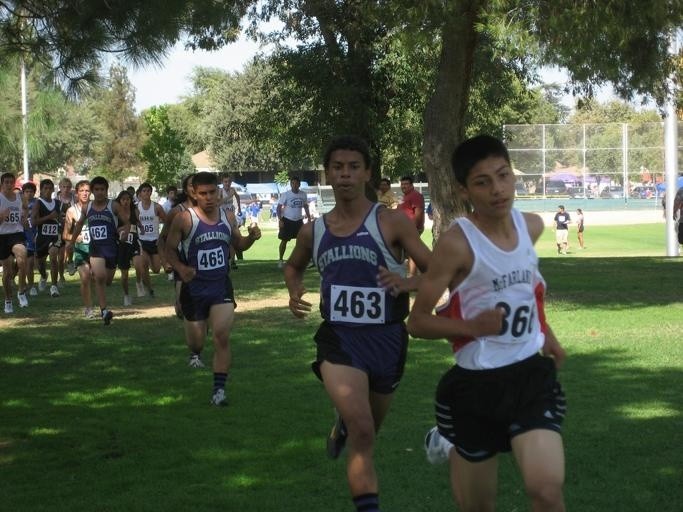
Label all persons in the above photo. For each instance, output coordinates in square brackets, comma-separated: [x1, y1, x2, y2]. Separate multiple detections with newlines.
[269, 197, 278, 219]
[673, 187, 682, 242]
[588, 179, 657, 199]
[406, 135, 568, 511]
[576, 208, 585, 249]
[284, 133, 433, 511]
[426, 204, 433, 220]
[221, 173, 242, 269]
[163, 172, 262, 405]
[236, 193, 262, 230]
[397, 176, 425, 279]
[376, 178, 394, 208]
[277, 176, 312, 262]
[0, 173, 197, 325]
[554, 205, 571, 254]
[662, 192, 667, 219]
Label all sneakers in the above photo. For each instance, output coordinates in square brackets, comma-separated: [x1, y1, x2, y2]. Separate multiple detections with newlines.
[425, 424, 448, 469]
[30, 273, 67, 297]
[277, 260, 283, 268]
[326, 407, 347, 459]
[4, 300, 13, 314]
[136, 284, 145, 297]
[123, 294, 132, 307]
[67, 260, 76, 275]
[102, 309, 112, 326]
[230, 263, 237, 269]
[18, 292, 28, 307]
[212, 388, 228, 407]
[190, 352, 200, 368]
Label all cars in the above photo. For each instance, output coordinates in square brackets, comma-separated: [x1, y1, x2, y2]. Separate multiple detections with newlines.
[12, 179, 39, 195]
[512, 176, 662, 198]
[216, 181, 323, 228]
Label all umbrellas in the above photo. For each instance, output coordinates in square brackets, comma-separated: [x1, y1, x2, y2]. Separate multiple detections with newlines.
[583, 175, 612, 183]
[549, 172, 583, 184]
[655, 176, 682, 191]
